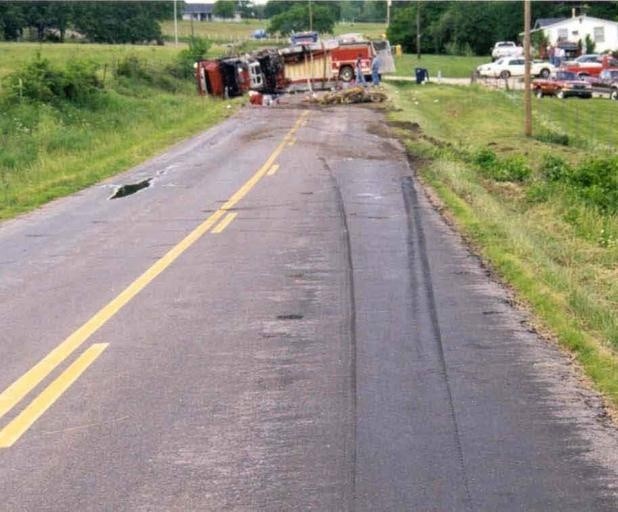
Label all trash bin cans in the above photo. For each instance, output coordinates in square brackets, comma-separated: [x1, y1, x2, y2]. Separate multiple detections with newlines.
[416, 68, 425, 83]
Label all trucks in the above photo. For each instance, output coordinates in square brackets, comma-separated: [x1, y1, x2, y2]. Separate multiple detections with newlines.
[188, 31, 398, 101]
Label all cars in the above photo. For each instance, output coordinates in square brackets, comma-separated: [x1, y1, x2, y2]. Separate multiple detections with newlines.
[475, 37, 618, 101]
[252, 30, 268, 38]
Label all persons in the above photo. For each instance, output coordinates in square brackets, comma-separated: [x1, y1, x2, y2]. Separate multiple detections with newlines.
[548, 44, 555, 64]
[553, 45, 562, 68]
[598, 52, 610, 81]
[367, 52, 382, 87]
[353, 53, 366, 84]
